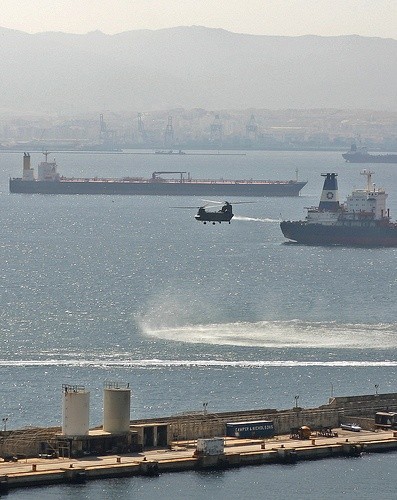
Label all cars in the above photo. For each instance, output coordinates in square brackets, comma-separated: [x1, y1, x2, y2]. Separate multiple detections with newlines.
[340, 423, 362, 431]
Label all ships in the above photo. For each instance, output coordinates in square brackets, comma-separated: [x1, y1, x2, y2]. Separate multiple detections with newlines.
[279, 170, 397, 248]
[8, 153, 307, 199]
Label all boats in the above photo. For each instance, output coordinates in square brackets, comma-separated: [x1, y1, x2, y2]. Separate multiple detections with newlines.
[341, 145, 397, 163]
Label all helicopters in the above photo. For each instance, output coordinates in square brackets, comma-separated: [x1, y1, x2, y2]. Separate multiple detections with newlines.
[169, 200, 256, 226]
